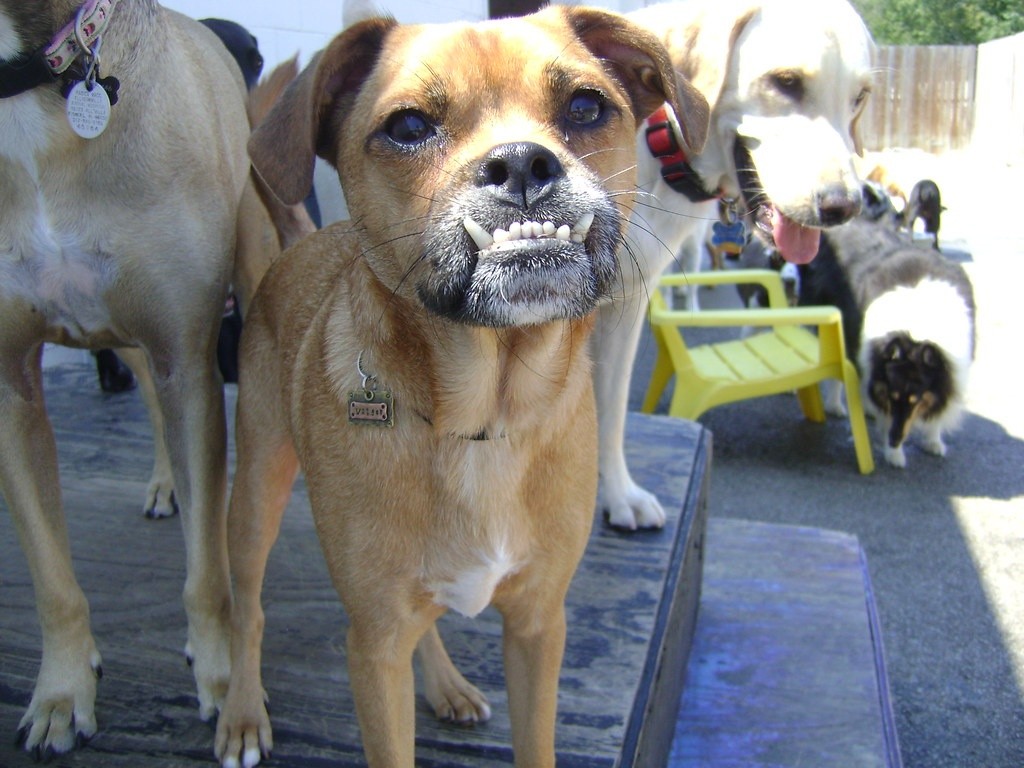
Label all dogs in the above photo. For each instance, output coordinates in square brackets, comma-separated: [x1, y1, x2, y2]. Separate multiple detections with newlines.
[215, 5, 711, 768]
[0, 0, 286, 751]
[826, 179, 977, 469]
[592, 0, 882, 532]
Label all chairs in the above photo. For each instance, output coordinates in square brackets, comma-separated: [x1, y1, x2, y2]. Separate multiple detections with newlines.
[649, 270, 878, 478]
